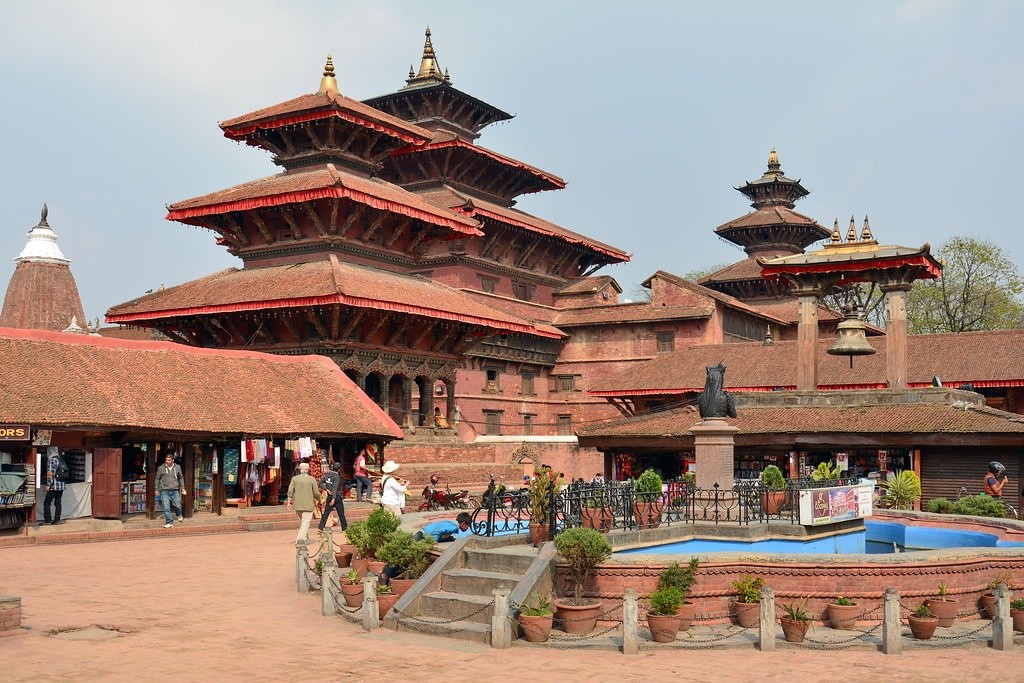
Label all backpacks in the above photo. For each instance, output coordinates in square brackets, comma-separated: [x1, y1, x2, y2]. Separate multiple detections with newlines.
[50, 456, 69, 482]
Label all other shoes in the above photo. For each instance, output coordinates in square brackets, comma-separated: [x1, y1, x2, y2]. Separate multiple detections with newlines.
[164, 523, 174, 528]
[178, 513, 183, 521]
[379, 573, 390, 587]
[39, 522, 50, 526]
[51, 521, 59, 525]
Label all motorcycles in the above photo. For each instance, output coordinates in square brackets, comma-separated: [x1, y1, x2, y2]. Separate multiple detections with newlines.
[419, 473, 530, 512]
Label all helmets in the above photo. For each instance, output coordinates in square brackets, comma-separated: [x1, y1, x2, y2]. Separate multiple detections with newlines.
[430, 475, 439, 483]
[989, 462, 1005, 473]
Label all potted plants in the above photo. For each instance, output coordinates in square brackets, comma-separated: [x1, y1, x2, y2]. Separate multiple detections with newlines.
[508, 587, 557, 643]
[778, 593, 816, 643]
[315, 502, 437, 620]
[926, 578, 960, 627]
[731, 573, 767, 627]
[759, 465, 787, 515]
[552, 525, 614, 636]
[1010, 597, 1024, 632]
[980, 570, 1014, 619]
[659, 555, 700, 631]
[644, 584, 689, 644]
[908, 604, 938, 639]
[785, 479, 800, 510]
[581, 488, 613, 533]
[629, 469, 663, 530]
[527, 463, 566, 547]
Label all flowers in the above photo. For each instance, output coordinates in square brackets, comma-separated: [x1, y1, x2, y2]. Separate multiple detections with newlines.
[837, 595, 852, 606]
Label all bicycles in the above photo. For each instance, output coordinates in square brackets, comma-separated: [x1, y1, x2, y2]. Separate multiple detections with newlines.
[955, 487, 1018, 519]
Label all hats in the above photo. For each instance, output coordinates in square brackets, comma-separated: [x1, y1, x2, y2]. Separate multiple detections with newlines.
[381, 461, 399, 473]
[297, 463, 311, 469]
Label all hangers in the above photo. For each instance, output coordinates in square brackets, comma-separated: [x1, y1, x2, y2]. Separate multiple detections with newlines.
[242, 433, 307, 448]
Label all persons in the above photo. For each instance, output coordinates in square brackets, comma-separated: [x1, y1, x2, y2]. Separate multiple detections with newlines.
[433, 407, 447, 428]
[154, 454, 187, 529]
[523, 465, 604, 486]
[39, 446, 64, 526]
[287, 463, 322, 545]
[381, 461, 410, 518]
[319, 463, 347, 534]
[837, 471, 852, 485]
[858, 470, 879, 509]
[984, 461, 1008, 501]
[378, 513, 472, 586]
[352, 447, 373, 503]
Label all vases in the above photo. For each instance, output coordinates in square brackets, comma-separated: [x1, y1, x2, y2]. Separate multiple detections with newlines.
[826, 600, 860, 631]
